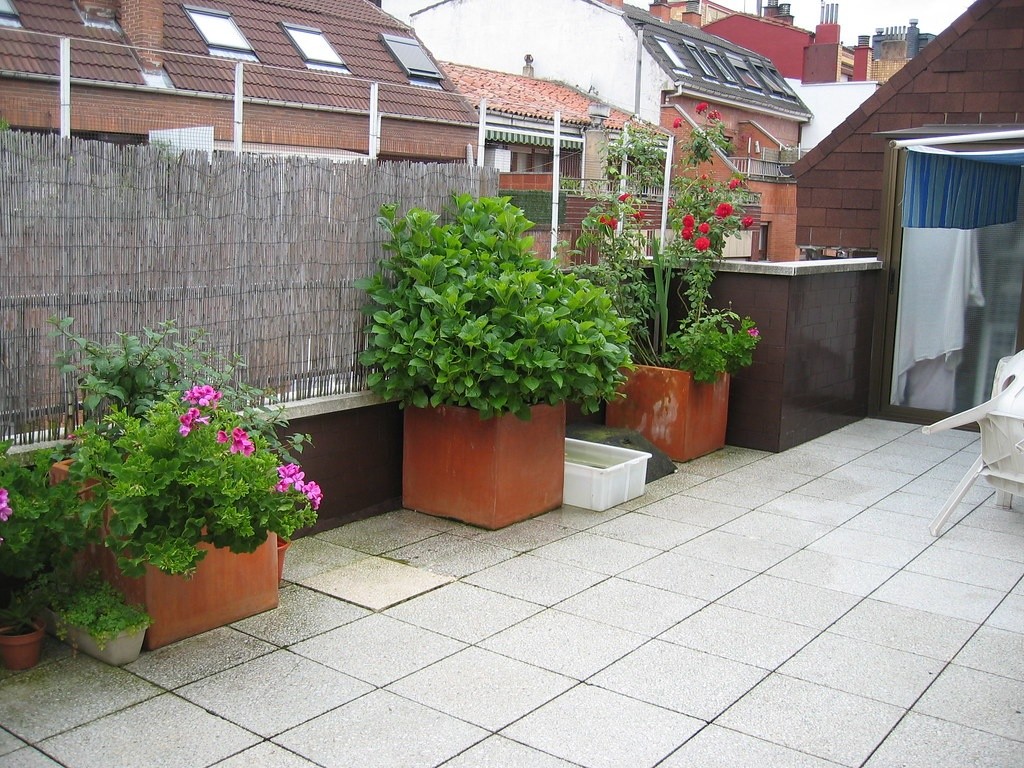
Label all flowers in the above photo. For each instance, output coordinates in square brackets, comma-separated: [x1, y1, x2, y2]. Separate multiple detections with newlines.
[575, 102, 762, 384]
[47, 311, 324, 582]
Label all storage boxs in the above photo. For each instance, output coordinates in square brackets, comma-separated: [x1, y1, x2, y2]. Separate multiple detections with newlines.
[564, 437, 653, 512]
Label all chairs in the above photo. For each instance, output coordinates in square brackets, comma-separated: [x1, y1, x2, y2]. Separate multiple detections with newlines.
[921, 349, 1024, 537]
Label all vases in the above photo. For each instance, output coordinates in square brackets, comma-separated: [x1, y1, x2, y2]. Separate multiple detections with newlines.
[48, 460, 280, 650]
[606, 363, 729, 463]
[277, 534, 292, 584]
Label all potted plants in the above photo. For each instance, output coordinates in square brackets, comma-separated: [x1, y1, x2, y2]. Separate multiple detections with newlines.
[349, 189, 642, 531]
[0, 589, 47, 670]
[33, 568, 156, 667]
